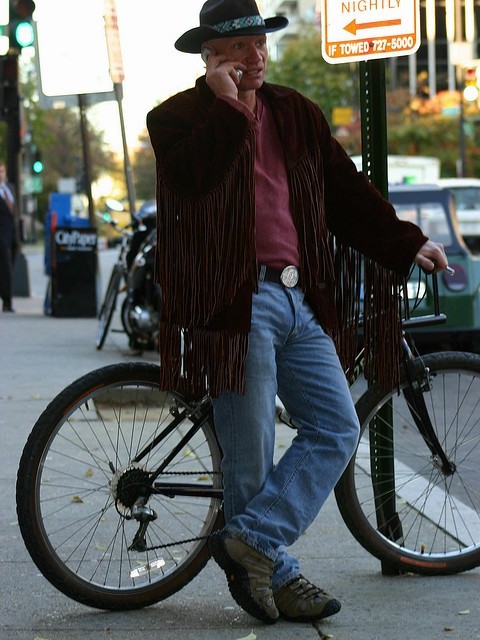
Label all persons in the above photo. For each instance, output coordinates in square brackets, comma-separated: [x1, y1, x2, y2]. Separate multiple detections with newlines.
[0, 164, 21, 310]
[146, 0, 449, 624]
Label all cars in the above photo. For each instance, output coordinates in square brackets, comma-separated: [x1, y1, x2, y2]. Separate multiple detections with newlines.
[350, 183, 480, 336]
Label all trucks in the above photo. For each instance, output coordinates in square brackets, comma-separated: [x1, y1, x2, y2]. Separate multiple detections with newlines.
[349, 156, 441, 236]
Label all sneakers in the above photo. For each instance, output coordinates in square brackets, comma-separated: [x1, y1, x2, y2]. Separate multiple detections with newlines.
[208, 526, 280, 624]
[272, 574, 341, 622]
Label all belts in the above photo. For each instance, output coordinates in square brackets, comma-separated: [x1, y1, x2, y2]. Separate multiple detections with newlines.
[257, 265, 300, 288]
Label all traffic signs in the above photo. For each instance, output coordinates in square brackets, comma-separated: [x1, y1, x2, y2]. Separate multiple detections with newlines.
[321, 0, 421, 65]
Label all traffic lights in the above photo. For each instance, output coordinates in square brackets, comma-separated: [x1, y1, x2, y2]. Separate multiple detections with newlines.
[29, 150, 44, 177]
[9, 0, 36, 48]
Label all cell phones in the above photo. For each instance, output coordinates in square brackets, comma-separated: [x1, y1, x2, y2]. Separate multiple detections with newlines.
[201, 48, 244, 81]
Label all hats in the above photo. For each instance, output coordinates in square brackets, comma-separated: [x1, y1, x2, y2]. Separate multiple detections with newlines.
[174, 0, 288, 53]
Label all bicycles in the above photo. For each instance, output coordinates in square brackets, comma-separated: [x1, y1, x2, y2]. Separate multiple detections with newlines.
[96, 212, 162, 350]
[15, 259, 480, 611]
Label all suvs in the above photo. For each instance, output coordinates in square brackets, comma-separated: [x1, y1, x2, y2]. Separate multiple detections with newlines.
[397, 176, 479, 242]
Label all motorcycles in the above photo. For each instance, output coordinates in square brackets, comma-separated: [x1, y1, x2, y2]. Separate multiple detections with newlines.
[105, 198, 162, 337]
